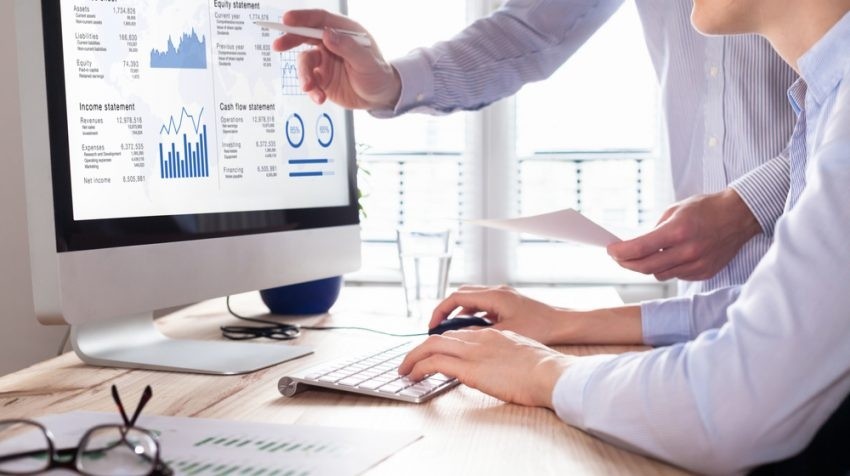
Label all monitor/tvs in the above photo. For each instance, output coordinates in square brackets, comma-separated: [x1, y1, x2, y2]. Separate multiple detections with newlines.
[14, 0, 361, 376]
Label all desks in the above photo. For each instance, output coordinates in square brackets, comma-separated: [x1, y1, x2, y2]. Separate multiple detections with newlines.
[0, 285, 748, 476]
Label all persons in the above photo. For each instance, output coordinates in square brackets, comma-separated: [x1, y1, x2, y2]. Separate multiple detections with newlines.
[273, 1, 849, 476]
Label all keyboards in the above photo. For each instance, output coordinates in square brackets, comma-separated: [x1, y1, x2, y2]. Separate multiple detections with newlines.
[276, 340, 461, 405]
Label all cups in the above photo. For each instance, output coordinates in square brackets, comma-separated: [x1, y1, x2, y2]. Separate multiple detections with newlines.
[396, 225, 451, 322]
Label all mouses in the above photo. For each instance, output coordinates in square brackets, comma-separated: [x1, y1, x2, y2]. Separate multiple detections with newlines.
[427, 318, 494, 336]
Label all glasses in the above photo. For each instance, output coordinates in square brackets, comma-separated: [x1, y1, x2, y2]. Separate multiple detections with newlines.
[0, 387, 174, 476]
